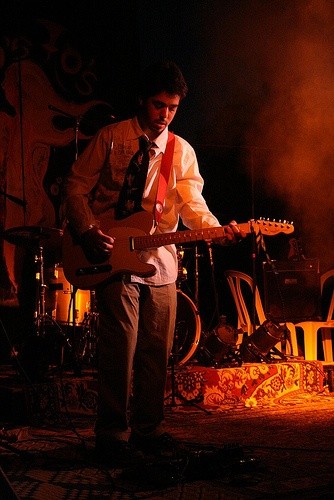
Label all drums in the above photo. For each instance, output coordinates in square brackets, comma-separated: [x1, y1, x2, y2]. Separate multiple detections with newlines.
[29, 264, 91, 331]
[170, 287, 202, 367]
[58, 311, 99, 368]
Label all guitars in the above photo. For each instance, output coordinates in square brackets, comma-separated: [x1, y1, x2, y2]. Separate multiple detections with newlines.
[62, 207, 297, 291]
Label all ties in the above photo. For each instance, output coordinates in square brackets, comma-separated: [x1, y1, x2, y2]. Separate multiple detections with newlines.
[123, 136, 156, 211]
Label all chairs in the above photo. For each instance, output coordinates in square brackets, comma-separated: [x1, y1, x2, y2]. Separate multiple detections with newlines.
[223, 269, 334, 364]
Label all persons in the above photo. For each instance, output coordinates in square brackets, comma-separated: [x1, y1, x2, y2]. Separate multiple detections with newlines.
[67, 59, 249, 467]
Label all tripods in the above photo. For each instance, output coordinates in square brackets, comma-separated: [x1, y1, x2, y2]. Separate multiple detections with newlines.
[161, 331, 214, 416]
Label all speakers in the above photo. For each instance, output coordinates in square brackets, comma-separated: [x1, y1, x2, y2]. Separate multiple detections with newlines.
[260, 257, 323, 323]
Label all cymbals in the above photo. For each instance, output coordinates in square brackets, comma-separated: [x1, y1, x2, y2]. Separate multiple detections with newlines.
[0, 226, 64, 251]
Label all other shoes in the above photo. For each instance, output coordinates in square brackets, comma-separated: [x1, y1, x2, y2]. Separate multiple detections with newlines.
[110, 431, 182, 465]
